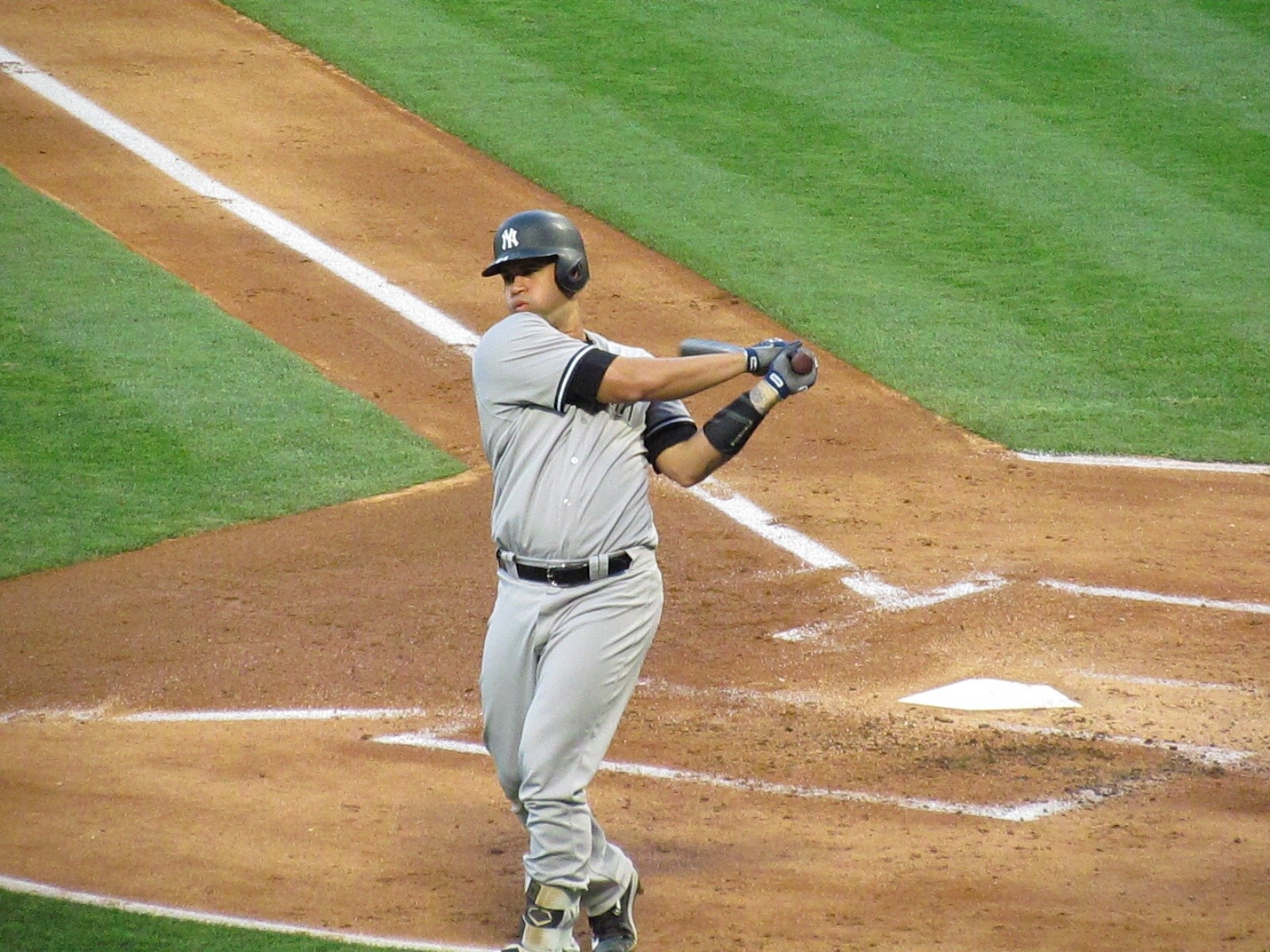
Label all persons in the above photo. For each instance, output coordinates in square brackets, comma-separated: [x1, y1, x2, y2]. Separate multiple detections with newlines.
[471, 208, 819, 952]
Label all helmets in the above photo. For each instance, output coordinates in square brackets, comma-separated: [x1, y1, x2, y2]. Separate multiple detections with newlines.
[481, 209, 590, 294]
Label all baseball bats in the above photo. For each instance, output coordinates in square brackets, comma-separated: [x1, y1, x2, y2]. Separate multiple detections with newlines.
[679, 339, 813, 375]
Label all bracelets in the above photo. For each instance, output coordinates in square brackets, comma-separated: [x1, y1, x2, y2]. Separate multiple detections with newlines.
[750, 380, 780, 416]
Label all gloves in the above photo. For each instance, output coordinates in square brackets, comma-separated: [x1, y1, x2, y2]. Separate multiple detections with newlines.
[745, 337, 789, 378]
[765, 340, 818, 400]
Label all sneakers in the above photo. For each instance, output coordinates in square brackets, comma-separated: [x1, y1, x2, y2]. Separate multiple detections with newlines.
[587, 867, 639, 952]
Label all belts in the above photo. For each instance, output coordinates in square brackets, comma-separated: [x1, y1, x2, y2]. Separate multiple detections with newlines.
[498, 553, 632, 588]
